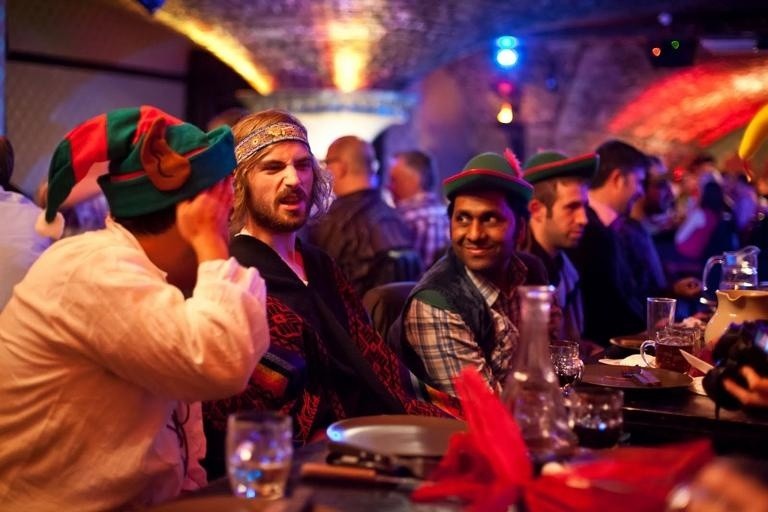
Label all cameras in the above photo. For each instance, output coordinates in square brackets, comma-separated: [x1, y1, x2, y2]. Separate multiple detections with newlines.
[701, 319, 768, 411]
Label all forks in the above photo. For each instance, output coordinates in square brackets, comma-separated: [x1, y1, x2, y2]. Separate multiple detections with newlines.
[620, 365, 661, 386]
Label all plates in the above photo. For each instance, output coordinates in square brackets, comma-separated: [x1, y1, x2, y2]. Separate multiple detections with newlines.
[608, 334, 647, 352]
[326, 412, 470, 461]
[575, 362, 696, 394]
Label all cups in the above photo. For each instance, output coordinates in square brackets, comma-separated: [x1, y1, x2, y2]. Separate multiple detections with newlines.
[539, 335, 625, 451]
[638, 297, 702, 374]
[225, 411, 295, 505]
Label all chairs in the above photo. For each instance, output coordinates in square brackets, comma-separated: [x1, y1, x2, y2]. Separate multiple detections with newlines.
[358, 280, 414, 340]
[364, 246, 426, 294]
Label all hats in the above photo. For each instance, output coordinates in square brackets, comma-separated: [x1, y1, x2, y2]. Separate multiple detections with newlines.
[35, 106, 236, 242]
[442, 152, 533, 206]
[520, 152, 600, 183]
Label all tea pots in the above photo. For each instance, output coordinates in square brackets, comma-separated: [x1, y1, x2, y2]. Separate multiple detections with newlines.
[702, 245, 761, 293]
[703, 288, 768, 349]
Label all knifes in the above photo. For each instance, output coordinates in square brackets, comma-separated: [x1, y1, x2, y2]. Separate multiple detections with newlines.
[679, 348, 714, 374]
[299, 462, 424, 489]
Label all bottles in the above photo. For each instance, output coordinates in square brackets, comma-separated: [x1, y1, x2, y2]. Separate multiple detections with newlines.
[505, 284, 577, 468]
